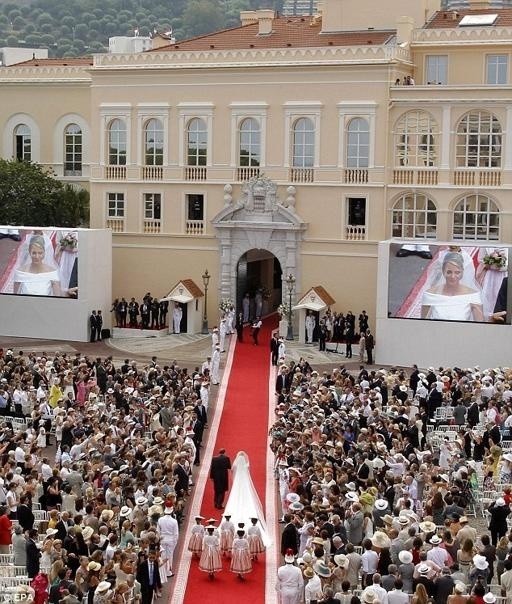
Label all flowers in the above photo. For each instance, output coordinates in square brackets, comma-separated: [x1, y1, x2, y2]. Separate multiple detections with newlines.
[220, 300, 233, 313]
[59, 233, 77, 248]
[483, 251, 505, 267]
[278, 304, 294, 320]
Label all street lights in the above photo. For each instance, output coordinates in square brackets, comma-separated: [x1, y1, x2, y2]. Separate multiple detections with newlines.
[201, 268, 211, 335]
[285, 273, 296, 340]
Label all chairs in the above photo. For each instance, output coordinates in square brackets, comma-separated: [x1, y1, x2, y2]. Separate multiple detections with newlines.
[354, 545, 364, 584]
[426, 407, 512, 527]
[0, 417, 49, 604]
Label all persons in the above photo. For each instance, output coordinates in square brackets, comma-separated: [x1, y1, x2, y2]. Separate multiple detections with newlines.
[68, 256, 79, 298]
[0, 228, 22, 241]
[407, 75, 414, 84]
[436, 246, 476, 280]
[305, 307, 374, 365]
[236, 289, 264, 346]
[25, 230, 54, 258]
[476, 246, 507, 315]
[418, 250, 506, 321]
[270, 333, 286, 366]
[276, 357, 511, 604]
[91, 293, 183, 342]
[395, 244, 432, 259]
[395, 77, 400, 85]
[187, 448, 273, 580]
[56, 231, 78, 289]
[402, 76, 407, 84]
[1, 347, 210, 604]
[490, 276, 508, 323]
[8, 233, 77, 297]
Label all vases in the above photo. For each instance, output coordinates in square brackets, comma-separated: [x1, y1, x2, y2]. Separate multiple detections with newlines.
[279, 320, 288, 337]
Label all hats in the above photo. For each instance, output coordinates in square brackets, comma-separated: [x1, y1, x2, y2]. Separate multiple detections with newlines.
[274, 359, 512, 603]
[2, 347, 216, 599]
[220, 510, 260, 533]
